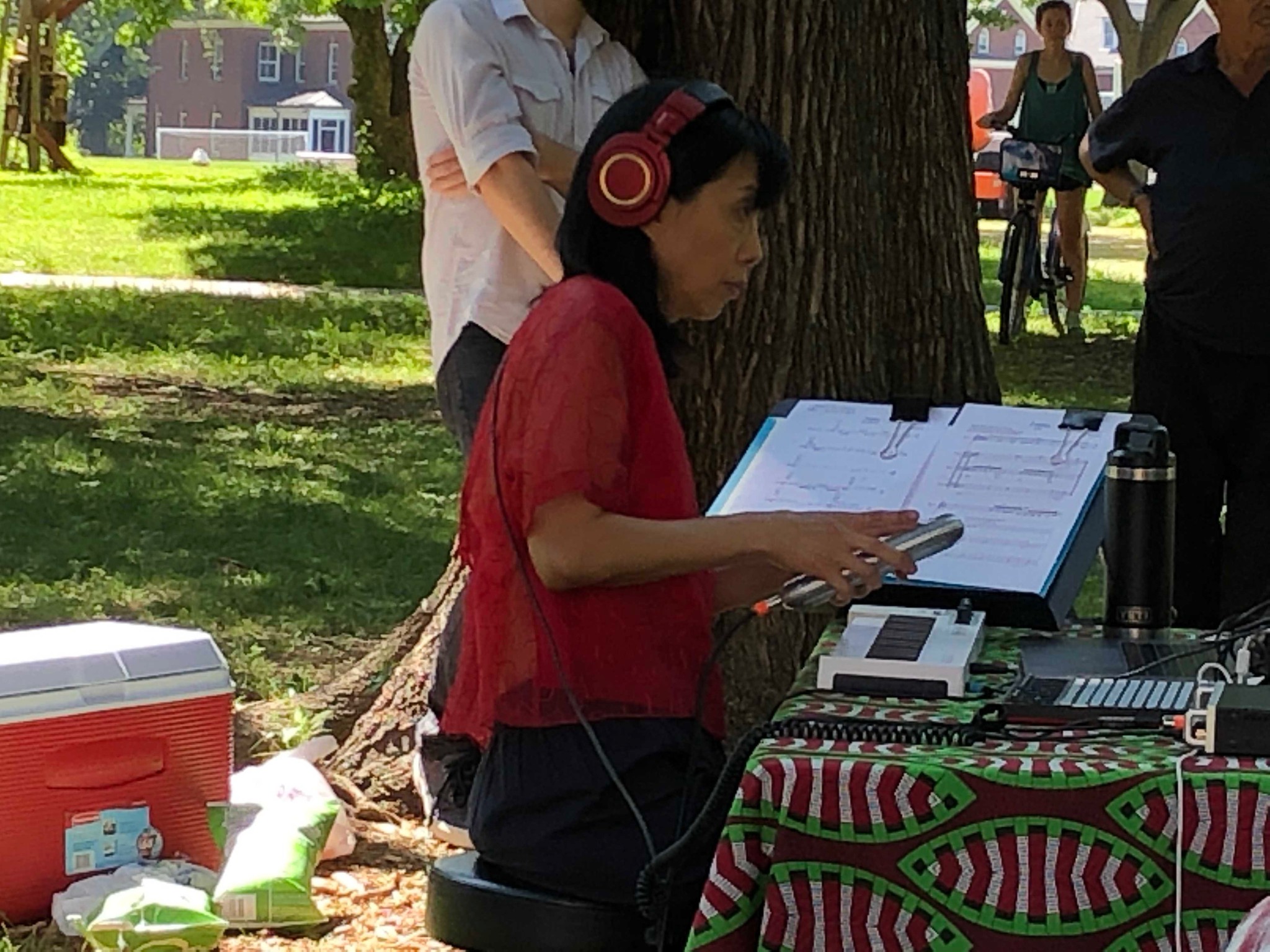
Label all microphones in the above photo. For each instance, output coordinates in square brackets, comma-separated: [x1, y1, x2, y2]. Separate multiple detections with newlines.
[778, 514, 966, 607]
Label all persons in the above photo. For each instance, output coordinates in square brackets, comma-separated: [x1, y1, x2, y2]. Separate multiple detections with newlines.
[1075, 0, 1270, 625]
[976, 0, 1104, 339]
[441, 73, 921, 952]
[409, 0, 648, 847]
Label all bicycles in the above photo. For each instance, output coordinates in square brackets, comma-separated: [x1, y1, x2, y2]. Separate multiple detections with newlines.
[985, 119, 1088, 344]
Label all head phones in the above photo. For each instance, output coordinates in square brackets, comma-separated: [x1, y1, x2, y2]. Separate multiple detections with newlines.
[584, 80, 734, 228]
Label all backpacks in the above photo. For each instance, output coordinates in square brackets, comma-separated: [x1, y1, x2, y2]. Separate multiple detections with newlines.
[999, 138, 1064, 187]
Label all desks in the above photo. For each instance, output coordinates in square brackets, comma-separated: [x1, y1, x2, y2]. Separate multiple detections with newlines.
[685, 606, 1270, 952]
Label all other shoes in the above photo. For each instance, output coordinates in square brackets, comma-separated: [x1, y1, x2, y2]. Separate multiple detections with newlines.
[1065, 312, 1084, 339]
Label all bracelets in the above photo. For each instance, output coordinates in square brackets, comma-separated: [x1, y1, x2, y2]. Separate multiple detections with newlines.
[1129, 190, 1141, 207]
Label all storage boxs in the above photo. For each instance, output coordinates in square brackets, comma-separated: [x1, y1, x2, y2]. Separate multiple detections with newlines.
[0, 620, 237, 932]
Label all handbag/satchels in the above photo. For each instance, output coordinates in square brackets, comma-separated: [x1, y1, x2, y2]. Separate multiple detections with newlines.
[227, 750, 355, 861]
[54, 856, 221, 936]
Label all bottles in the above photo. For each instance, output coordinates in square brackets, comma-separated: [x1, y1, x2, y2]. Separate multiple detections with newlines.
[1103, 421, 1176, 640]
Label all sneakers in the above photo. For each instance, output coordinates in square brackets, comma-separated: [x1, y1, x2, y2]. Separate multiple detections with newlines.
[411, 710, 486, 849]
